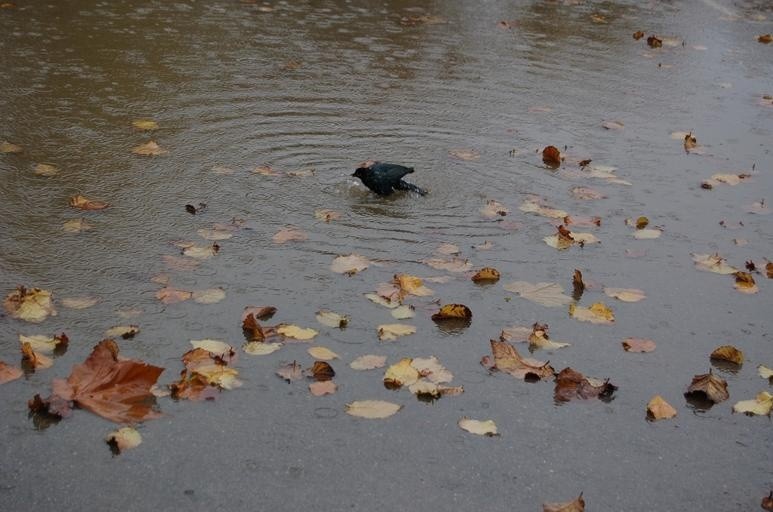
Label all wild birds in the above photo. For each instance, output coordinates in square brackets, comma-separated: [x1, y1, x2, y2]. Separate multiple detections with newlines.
[350, 161, 427, 200]
